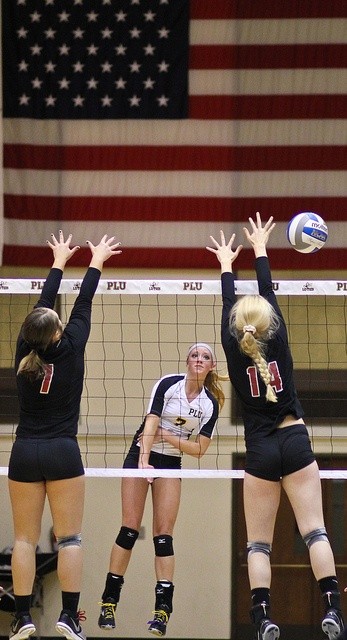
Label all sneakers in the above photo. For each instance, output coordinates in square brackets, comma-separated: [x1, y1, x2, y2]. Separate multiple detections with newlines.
[98, 597, 117, 630]
[256, 618, 281, 640]
[147, 603, 170, 636]
[8, 613, 37, 640]
[55, 608, 87, 640]
[321, 607, 345, 640]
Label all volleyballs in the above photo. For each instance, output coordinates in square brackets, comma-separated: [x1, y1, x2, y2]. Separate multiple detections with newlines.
[285, 212, 328, 254]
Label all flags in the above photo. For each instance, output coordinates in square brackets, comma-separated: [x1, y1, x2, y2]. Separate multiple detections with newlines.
[0, 2, 346, 271]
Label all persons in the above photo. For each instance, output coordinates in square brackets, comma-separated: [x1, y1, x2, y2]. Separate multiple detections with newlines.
[97, 343, 227, 638]
[204, 212, 346, 639]
[7, 229, 123, 639]
